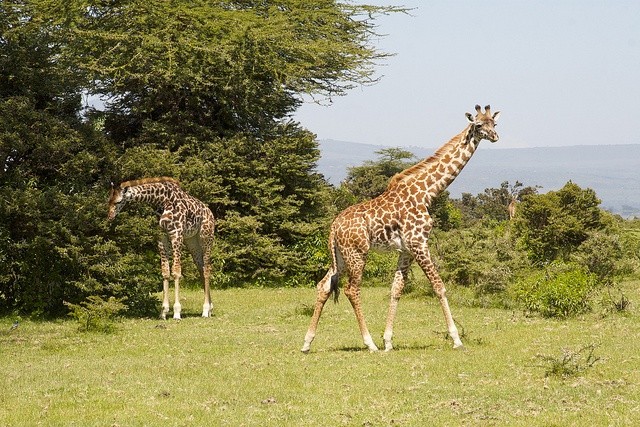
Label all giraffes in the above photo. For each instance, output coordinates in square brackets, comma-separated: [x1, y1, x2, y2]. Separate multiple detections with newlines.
[301, 104, 501, 353]
[107, 176, 215, 322]
